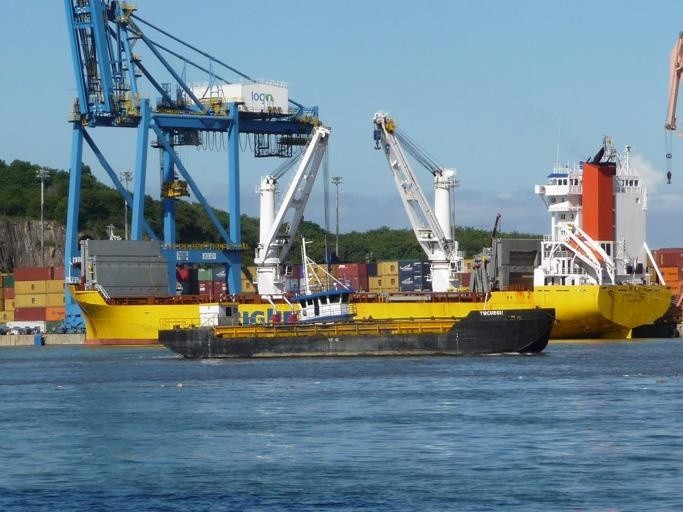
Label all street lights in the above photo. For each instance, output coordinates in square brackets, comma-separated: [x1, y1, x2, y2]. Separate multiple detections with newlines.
[330, 177, 342, 257]
[119, 170, 132, 240]
[35, 169, 50, 269]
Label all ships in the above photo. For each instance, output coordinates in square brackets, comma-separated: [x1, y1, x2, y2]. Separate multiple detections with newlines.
[70, 110, 673, 344]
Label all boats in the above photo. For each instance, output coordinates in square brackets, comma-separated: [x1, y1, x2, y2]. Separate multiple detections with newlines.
[157, 237, 555, 359]
